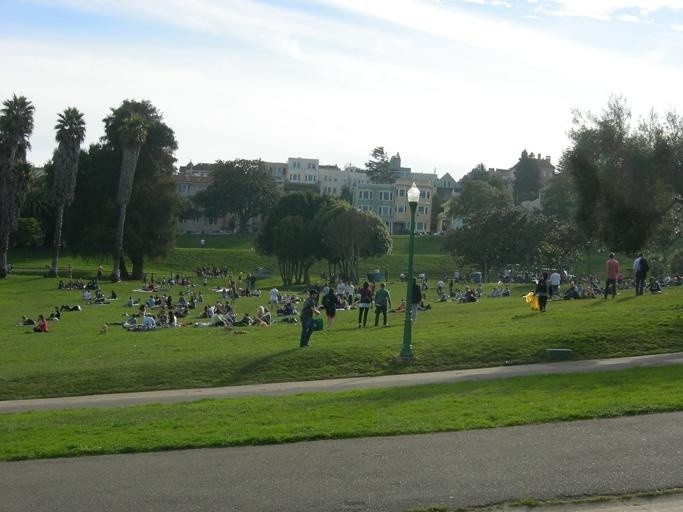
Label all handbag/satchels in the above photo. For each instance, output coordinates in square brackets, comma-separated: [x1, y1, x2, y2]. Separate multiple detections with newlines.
[309, 318, 323, 331]
[31, 326, 42, 332]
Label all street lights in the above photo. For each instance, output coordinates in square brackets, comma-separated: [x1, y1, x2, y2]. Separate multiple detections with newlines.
[400, 181, 421, 359]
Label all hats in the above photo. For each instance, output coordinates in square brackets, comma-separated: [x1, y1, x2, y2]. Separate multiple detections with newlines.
[308, 289, 319, 294]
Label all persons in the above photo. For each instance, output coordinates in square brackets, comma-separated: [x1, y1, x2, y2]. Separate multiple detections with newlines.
[410, 277, 422, 323]
[604, 251, 620, 299]
[44, 263, 110, 305]
[100, 264, 377, 335]
[373, 282, 392, 328]
[299, 289, 324, 349]
[631, 252, 649, 296]
[388, 263, 683, 314]
[19, 304, 81, 333]
